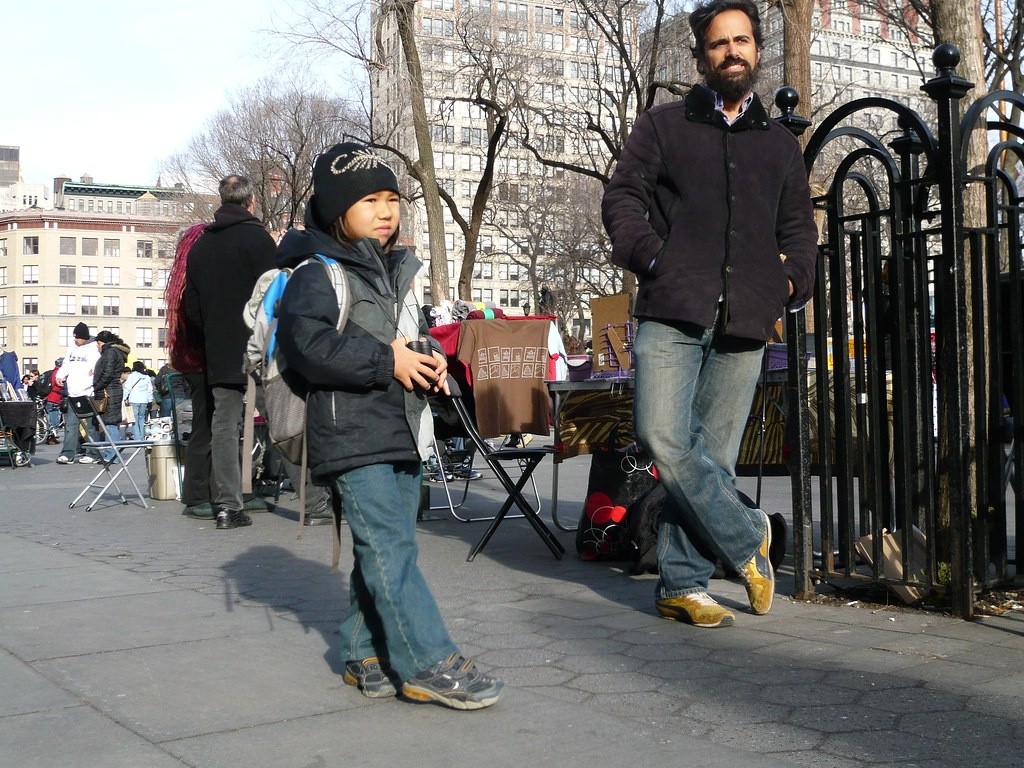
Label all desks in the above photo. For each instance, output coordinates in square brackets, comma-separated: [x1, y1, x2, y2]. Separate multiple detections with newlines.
[546, 367, 895, 540]
[429, 315, 556, 523]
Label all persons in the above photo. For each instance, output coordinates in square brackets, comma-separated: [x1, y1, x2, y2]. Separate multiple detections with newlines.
[274, 142, 504, 710]
[162, 174, 335, 529]
[0, 321, 191, 466]
[601, 0, 819, 628]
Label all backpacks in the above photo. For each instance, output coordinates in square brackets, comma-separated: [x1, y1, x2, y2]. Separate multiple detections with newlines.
[36, 369, 55, 396]
[243, 253, 350, 443]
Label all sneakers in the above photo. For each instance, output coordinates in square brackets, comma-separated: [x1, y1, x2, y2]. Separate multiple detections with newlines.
[733, 509, 774, 614]
[656, 586, 736, 628]
[430, 469, 453, 483]
[344, 655, 398, 698]
[402, 649, 503, 710]
[217, 508, 251, 530]
[453, 468, 482, 480]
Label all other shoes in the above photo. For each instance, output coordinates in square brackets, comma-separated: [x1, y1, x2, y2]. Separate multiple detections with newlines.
[187, 501, 218, 520]
[47, 437, 59, 444]
[79, 455, 94, 464]
[304, 502, 348, 526]
[97, 459, 116, 465]
[56, 455, 75, 464]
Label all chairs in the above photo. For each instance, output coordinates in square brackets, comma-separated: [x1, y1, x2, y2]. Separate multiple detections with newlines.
[66, 395, 154, 513]
[442, 374, 566, 563]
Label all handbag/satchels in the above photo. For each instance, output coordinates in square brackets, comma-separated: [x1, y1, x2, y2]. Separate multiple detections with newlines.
[160, 368, 169, 395]
[90, 396, 108, 412]
[125, 397, 129, 407]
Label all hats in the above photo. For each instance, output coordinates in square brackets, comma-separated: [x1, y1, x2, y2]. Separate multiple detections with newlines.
[313, 142, 401, 225]
[73, 322, 90, 340]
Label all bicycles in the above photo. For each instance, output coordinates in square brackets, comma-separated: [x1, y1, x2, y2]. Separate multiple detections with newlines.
[35, 399, 65, 445]
